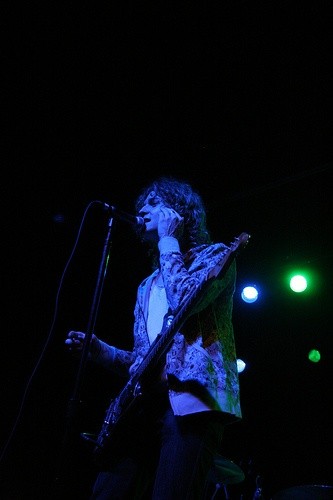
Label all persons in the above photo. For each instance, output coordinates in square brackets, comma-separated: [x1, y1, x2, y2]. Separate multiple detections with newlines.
[64, 179, 243, 500]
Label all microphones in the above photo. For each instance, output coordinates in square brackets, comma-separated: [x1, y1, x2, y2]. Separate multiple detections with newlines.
[96, 200, 144, 228]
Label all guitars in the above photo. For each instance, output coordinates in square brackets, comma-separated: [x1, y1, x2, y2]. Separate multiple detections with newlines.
[91, 231, 252, 453]
